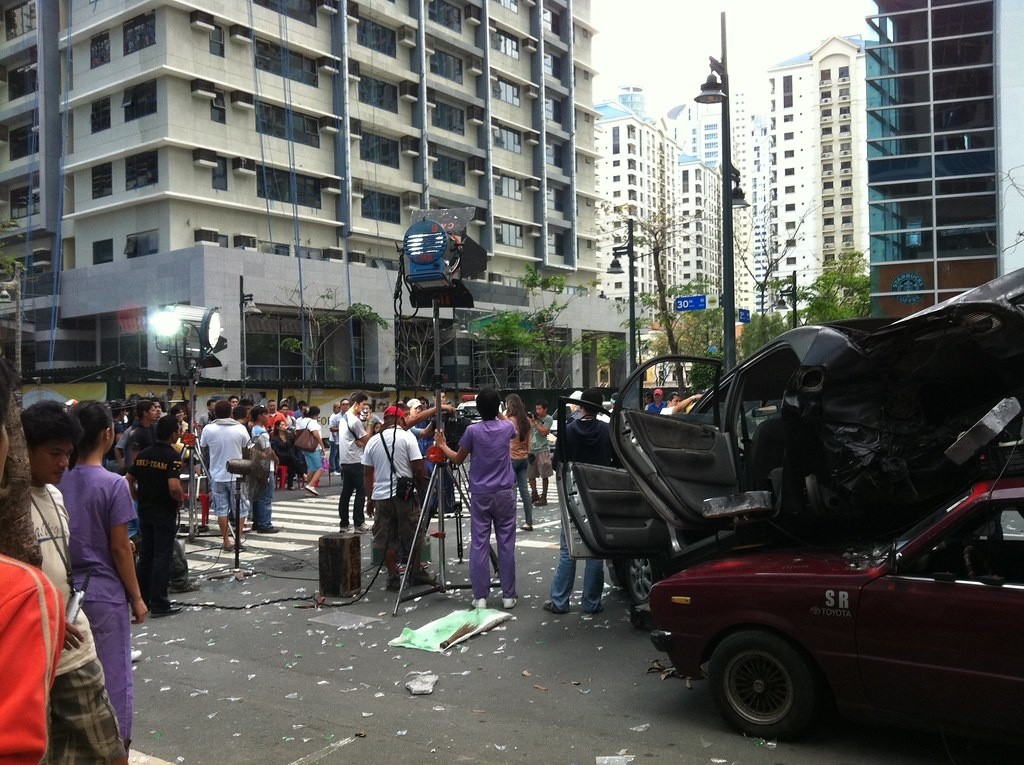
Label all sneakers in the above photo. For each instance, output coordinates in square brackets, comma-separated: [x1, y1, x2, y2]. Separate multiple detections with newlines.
[355, 523, 373, 533]
[339, 524, 354, 532]
[471, 597, 486, 609]
[501, 596, 517, 608]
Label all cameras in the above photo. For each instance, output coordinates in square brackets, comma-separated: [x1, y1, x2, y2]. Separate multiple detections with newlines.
[526, 411, 537, 420]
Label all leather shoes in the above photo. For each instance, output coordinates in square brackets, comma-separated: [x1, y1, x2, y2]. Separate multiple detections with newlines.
[169, 598, 177, 604]
[152, 604, 183, 618]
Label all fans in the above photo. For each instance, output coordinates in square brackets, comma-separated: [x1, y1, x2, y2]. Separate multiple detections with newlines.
[207, 436, 272, 581]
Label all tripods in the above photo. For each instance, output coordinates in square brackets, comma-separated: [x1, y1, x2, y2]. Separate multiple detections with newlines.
[391, 296, 518, 617]
[176, 361, 238, 545]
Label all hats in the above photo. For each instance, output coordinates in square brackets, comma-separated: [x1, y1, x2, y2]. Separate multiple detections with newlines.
[65, 399, 78, 405]
[406, 398, 423, 409]
[653, 388, 663, 395]
[383, 406, 407, 426]
[280, 397, 290, 406]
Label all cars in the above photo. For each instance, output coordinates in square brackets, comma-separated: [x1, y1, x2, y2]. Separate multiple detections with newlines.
[645, 472, 1024, 743]
[444, 394, 505, 444]
[549, 270, 1023, 624]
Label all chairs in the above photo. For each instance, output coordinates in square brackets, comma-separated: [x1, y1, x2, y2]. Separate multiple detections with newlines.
[275, 465, 301, 490]
[743, 420, 786, 486]
[183, 494, 210, 526]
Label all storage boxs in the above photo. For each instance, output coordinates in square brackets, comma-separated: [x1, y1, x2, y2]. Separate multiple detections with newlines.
[370, 537, 432, 565]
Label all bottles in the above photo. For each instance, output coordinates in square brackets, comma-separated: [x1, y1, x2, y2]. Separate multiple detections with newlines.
[285, 426, 294, 430]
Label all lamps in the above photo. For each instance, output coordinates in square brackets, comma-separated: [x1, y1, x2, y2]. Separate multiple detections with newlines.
[161, 305, 221, 349]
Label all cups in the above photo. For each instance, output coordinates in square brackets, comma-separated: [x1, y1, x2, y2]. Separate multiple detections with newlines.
[361, 409, 368, 418]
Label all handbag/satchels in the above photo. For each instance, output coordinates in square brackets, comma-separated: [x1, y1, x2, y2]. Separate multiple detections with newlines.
[397, 476, 415, 501]
[294, 421, 318, 452]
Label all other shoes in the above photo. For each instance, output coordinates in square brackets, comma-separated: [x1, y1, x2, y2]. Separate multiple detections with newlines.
[258, 527, 279, 533]
[530, 494, 547, 506]
[223, 542, 234, 551]
[131, 650, 141, 660]
[252, 523, 257, 530]
[387, 575, 401, 591]
[287, 486, 295, 490]
[521, 524, 534, 531]
[170, 581, 201, 593]
[304, 492, 316, 497]
[543, 600, 552, 611]
[305, 485, 319, 495]
[187, 576, 198, 582]
[409, 574, 437, 586]
[234, 544, 246, 552]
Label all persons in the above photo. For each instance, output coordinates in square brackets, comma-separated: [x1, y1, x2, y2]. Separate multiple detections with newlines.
[0, 394, 324, 765]
[329, 389, 705, 614]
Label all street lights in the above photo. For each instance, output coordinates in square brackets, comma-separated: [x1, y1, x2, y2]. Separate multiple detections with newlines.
[607, 218, 636, 389]
[691, 11, 750, 377]
[239, 274, 262, 401]
[778, 271, 798, 329]
[1, 261, 27, 392]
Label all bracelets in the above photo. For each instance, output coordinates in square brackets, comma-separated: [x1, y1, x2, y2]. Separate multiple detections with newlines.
[691, 395, 696, 402]
[366, 422, 372, 426]
[533, 422, 538, 426]
